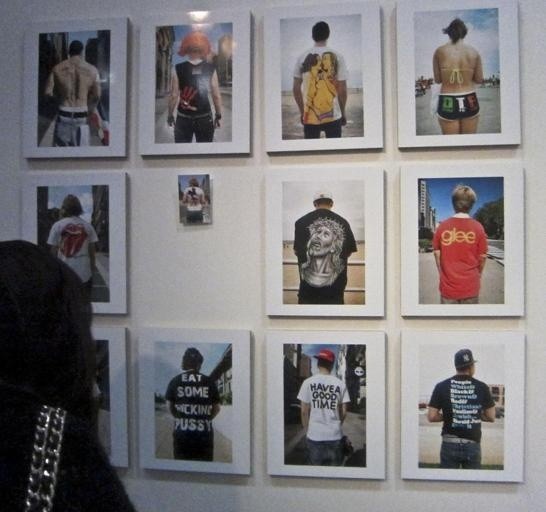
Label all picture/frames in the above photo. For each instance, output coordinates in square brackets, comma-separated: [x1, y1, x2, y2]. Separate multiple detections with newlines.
[268, 164, 386, 320]
[18, 168, 128, 315]
[264, 328, 392, 481]
[136, 7, 251, 157]
[399, 332, 525, 485]
[265, 0, 386, 154]
[136, 325, 251, 477]
[401, 161, 527, 317]
[86, 325, 126, 473]
[398, 0, 520, 152]
[27, 18, 129, 159]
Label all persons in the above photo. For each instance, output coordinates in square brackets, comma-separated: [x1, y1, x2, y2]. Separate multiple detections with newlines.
[289, 22, 351, 139]
[162, 346, 221, 459]
[0, 232, 141, 510]
[161, 31, 222, 143]
[290, 189, 359, 305]
[298, 216, 348, 287]
[431, 16, 487, 134]
[432, 183, 489, 305]
[43, 41, 107, 148]
[182, 176, 208, 227]
[426, 347, 496, 470]
[47, 194, 101, 305]
[298, 350, 349, 464]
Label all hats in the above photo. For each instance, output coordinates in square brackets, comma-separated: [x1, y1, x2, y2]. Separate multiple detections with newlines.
[454, 349, 476, 368]
[311, 190, 332, 202]
[313, 349, 334, 362]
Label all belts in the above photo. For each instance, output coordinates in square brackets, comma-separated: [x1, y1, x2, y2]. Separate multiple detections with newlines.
[58, 109, 88, 117]
